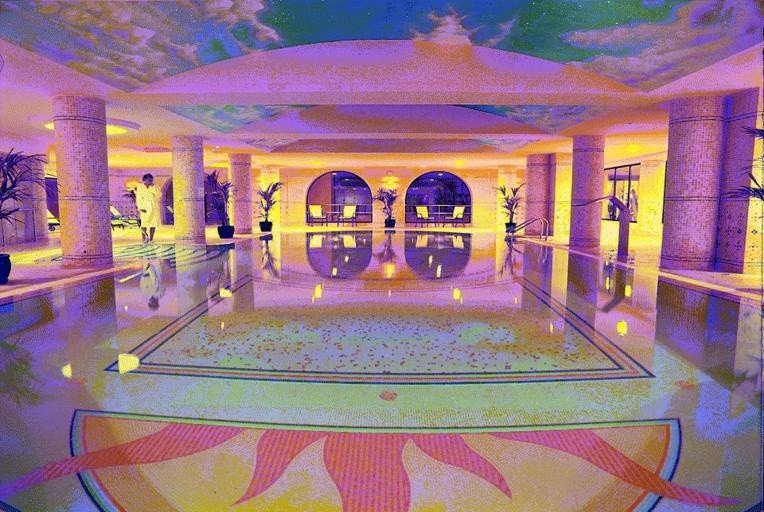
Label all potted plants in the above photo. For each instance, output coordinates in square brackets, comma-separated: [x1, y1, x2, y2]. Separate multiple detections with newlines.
[0, 148, 63, 285]
[498, 236, 523, 277]
[259, 234, 280, 280]
[372, 188, 398, 227]
[204, 170, 234, 239]
[374, 229, 398, 264]
[257, 181, 283, 231]
[494, 183, 526, 232]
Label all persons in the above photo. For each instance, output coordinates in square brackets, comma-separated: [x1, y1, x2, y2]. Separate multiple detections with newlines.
[135, 174, 163, 243]
[139, 259, 171, 312]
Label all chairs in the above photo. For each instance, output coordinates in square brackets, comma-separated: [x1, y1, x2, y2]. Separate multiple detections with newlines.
[414, 206, 438, 229]
[307, 204, 329, 227]
[111, 220, 126, 231]
[441, 207, 466, 228]
[109, 205, 140, 232]
[338, 205, 357, 227]
